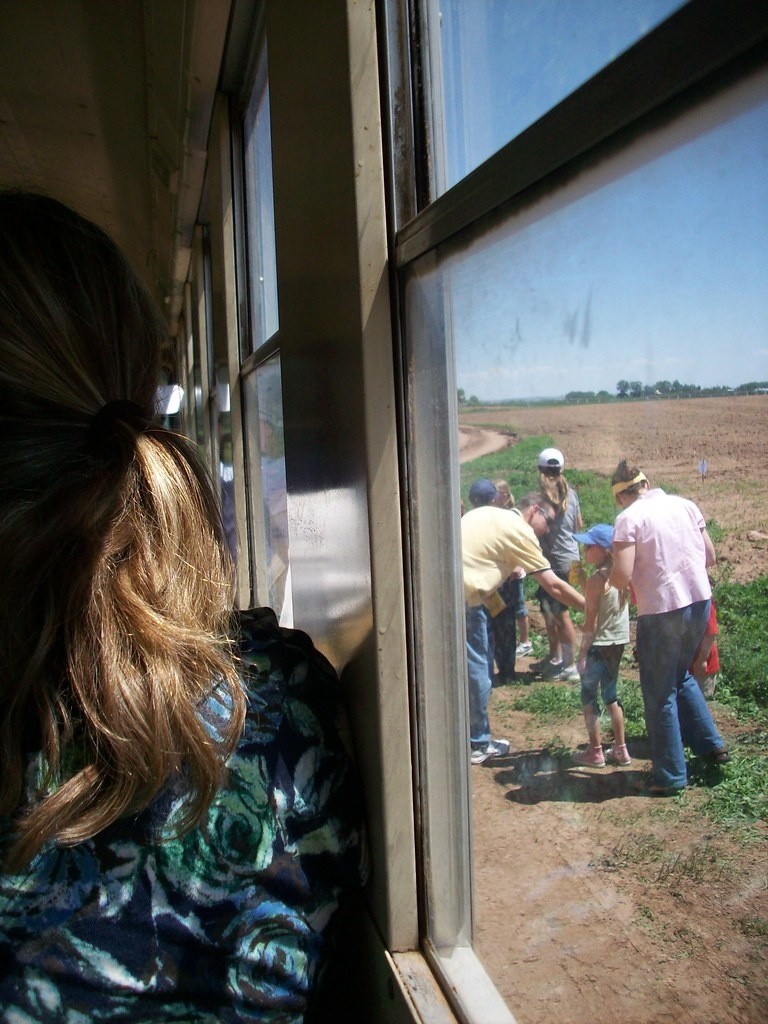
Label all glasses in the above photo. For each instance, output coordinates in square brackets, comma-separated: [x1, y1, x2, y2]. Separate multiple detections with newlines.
[538, 506, 556, 529]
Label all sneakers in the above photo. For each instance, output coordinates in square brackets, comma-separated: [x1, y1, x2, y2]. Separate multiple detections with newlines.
[602, 743, 632, 766]
[470, 738, 510, 764]
[572, 746, 607, 768]
[514, 642, 535, 657]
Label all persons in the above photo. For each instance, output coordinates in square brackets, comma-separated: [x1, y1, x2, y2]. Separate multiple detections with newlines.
[0, 189, 373, 1024]
[456, 446, 731, 796]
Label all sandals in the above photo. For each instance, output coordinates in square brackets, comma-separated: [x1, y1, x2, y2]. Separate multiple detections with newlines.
[709, 748, 731, 763]
[634, 776, 684, 793]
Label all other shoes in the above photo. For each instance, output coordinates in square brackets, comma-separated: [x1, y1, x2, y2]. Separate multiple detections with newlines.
[541, 660, 579, 681]
[529, 655, 552, 671]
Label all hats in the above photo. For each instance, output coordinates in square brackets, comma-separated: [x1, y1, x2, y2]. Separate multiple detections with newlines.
[572, 523, 615, 550]
[611, 471, 651, 508]
[469, 478, 502, 505]
[537, 448, 565, 469]
